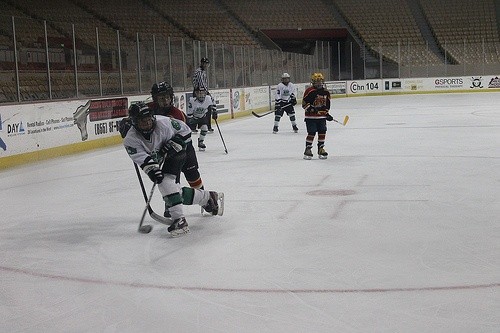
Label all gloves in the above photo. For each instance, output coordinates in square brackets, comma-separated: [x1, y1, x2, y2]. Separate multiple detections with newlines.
[326, 113, 333, 122]
[188, 118, 197, 130]
[290, 96, 297, 106]
[307, 106, 318, 115]
[147, 168, 164, 184]
[212, 111, 217, 119]
[160, 136, 184, 158]
[275, 102, 281, 112]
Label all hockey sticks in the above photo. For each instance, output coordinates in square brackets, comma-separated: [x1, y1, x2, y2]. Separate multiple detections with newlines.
[319, 111, 349, 126]
[214, 119, 228, 153]
[133, 161, 174, 225]
[252, 103, 291, 118]
[139, 149, 175, 233]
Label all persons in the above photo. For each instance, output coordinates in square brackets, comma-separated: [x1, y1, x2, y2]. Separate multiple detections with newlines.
[187, 87, 218, 151]
[301, 73, 333, 159]
[123, 102, 224, 237]
[192, 57, 214, 134]
[119, 81, 205, 217]
[273, 73, 299, 133]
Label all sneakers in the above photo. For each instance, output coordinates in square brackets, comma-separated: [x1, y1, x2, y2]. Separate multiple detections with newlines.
[191, 129, 198, 134]
[318, 149, 328, 159]
[208, 128, 214, 131]
[273, 126, 279, 134]
[292, 125, 299, 133]
[163, 202, 172, 219]
[204, 191, 224, 216]
[198, 138, 206, 152]
[303, 147, 314, 160]
[168, 217, 191, 238]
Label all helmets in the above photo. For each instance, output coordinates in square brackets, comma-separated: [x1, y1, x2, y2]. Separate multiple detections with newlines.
[194, 86, 207, 95]
[129, 102, 155, 128]
[201, 57, 209, 63]
[311, 73, 324, 82]
[152, 81, 174, 102]
[280, 73, 290, 78]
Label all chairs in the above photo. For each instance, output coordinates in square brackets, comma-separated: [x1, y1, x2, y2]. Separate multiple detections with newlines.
[0, 0, 500, 104]
